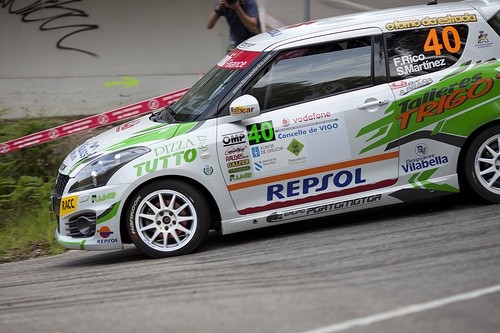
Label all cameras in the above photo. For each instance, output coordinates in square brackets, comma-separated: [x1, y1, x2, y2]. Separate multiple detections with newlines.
[224, 0, 238, 3]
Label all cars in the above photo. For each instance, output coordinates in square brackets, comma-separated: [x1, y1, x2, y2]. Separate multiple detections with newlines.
[50, 0, 500, 258]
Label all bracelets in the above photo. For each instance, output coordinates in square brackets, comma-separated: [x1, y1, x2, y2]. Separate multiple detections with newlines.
[214, 8, 221, 13]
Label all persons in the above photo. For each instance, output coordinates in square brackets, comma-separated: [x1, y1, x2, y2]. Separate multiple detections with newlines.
[207, 1, 263, 54]
[298, 43, 347, 104]
